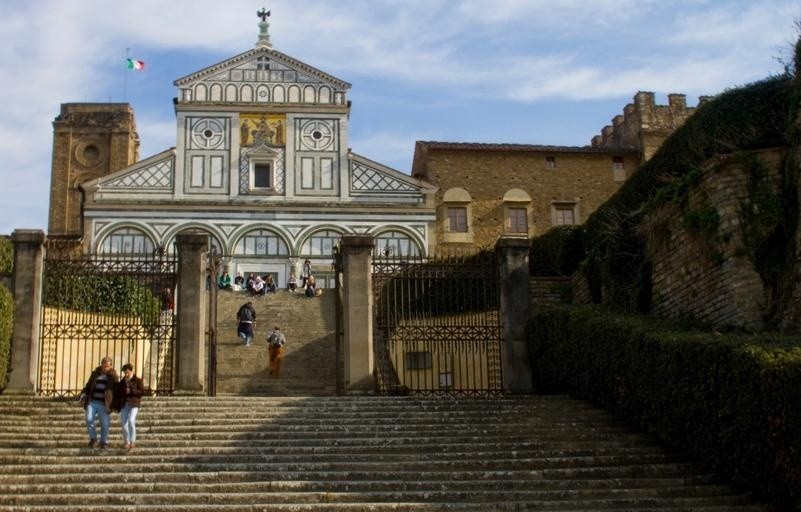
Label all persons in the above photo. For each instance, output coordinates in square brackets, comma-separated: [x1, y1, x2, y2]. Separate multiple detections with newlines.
[236, 302, 258, 345]
[77, 357, 119, 449]
[208, 267, 277, 297]
[299, 260, 312, 287]
[118, 363, 144, 448]
[242, 118, 284, 145]
[287, 273, 298, 293]
[267, 325, 285, 377]
[304, 274, 316, 297]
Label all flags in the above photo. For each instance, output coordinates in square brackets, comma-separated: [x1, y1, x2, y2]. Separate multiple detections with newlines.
[127, 58, 145, 70]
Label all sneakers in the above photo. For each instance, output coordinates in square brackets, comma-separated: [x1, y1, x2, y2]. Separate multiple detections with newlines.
[122, 442, 135, 448]
[88, 439, 108, 448]
